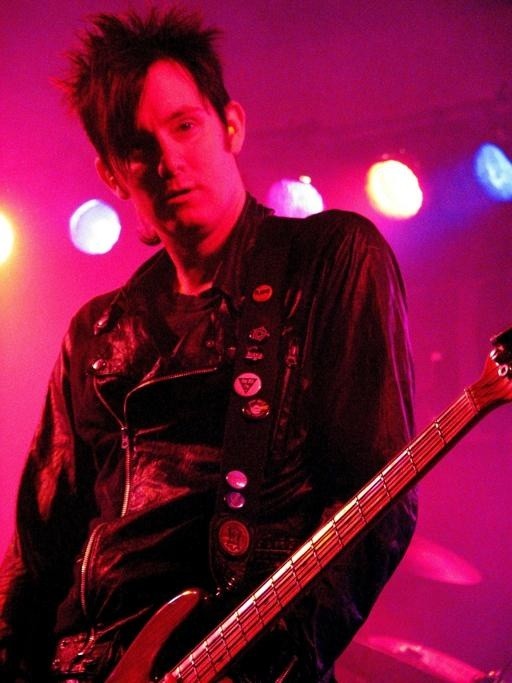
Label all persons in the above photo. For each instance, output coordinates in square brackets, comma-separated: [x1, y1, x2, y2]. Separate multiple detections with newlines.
[1, 2, 419, 683]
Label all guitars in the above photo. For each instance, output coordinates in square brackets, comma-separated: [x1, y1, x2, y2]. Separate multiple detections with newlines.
[99, 325, 512, 683]
